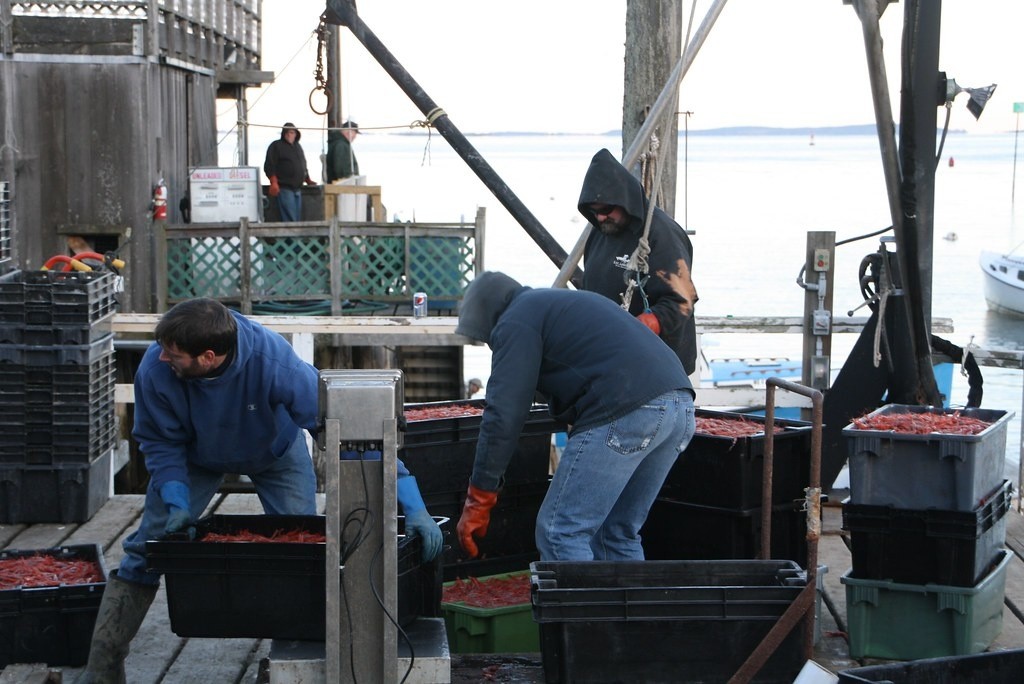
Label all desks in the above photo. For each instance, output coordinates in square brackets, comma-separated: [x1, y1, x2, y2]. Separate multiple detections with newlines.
[323, 184, 382, 222]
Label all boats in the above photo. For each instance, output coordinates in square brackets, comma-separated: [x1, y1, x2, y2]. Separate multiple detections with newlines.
[979, 250, 1024, 321]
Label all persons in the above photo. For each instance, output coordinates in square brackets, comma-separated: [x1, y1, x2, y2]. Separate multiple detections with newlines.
[263, 122, 317, 257]
[81, 296, 445, 684]
[452, 270, 699, 562]
[326, 121, 364, 217]
[576, 148, 699, 377]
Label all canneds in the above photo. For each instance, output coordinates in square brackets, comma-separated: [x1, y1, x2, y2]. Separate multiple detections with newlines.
[413, 292, 428, 317]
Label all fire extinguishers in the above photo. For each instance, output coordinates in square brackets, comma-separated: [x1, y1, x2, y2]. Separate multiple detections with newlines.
[153, 179, 169, 220]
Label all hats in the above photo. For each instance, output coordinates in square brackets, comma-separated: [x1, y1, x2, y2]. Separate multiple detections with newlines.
[342, 121, 362, 134]
[468, 378, 484, 388]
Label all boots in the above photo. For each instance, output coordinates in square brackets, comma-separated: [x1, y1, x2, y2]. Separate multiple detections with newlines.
[71, 568, 159, 683]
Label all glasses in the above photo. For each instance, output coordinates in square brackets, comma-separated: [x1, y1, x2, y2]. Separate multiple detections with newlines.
[584, 204, 618, 216]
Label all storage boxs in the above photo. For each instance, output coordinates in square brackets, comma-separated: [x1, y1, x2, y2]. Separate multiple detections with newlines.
[442, 569, 541, 653]
[430, 481, 551, 558]
[842, 480, 1013, 584]
[0, 269, 120, 524]
[0, 540, 110, 667]
[643, 494, 829, 558]
[400, 397, 562, 493]
[144, 511, 452, 639]
[658, 407, 824, 506]
[529, 556, 811, 684]
[843, 547, 1018, 658]
[843, 401, 1015, 507]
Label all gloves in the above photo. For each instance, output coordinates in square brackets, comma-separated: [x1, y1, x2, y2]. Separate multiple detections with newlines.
[269, 175, 280, 196]
[305, 178, 317, 186]
[635, 311, 661, 334]
[160, 480, 197, 540]
[395, 475, 444, 564]
[456, 484, 498, 558]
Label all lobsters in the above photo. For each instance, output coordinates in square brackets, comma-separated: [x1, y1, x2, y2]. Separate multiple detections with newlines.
[852, 410, 995, 434]
[440, 574, 530, 608]
[402, 405, 484, 421]
[691, 414, 783, 438]
[197, 526, 327, 543]
[0, 554, 99, 590]
